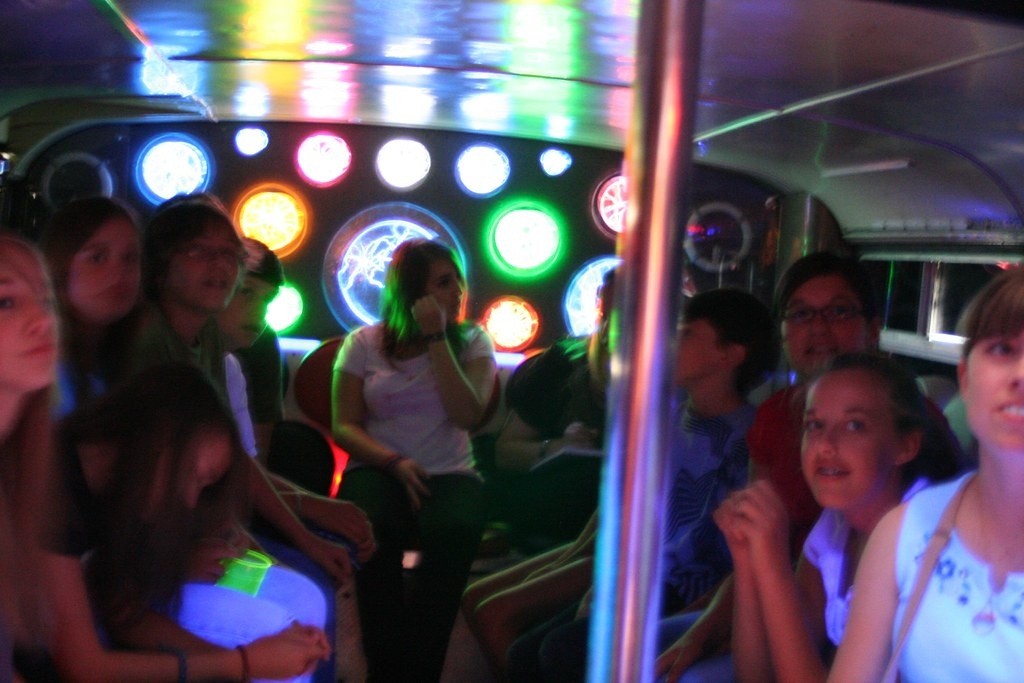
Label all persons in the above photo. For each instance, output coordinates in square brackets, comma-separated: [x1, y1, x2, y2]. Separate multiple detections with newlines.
[0, 192, 376, 683]
[443, 246, 1024, 683]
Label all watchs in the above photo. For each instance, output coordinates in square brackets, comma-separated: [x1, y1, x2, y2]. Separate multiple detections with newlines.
[331, 239, 496, 683]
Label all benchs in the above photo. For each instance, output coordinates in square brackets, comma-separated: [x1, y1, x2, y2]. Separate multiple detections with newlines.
[277, 338, 544, 567]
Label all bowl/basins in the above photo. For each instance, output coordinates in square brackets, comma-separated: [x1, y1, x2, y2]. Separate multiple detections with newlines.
[214, 544, 272, 599]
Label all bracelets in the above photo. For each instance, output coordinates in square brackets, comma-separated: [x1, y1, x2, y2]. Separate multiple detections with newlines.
[423, 331, 447, 345]
[383, 453, 403, 466]
[539, 438, 556, 465]
[158, 646, 187, 683]
[234, 644, 250, 683]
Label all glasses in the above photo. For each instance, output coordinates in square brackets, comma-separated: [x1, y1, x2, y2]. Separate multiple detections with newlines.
[780, 303, 865, 324]
[172, 241, 248, 263]
